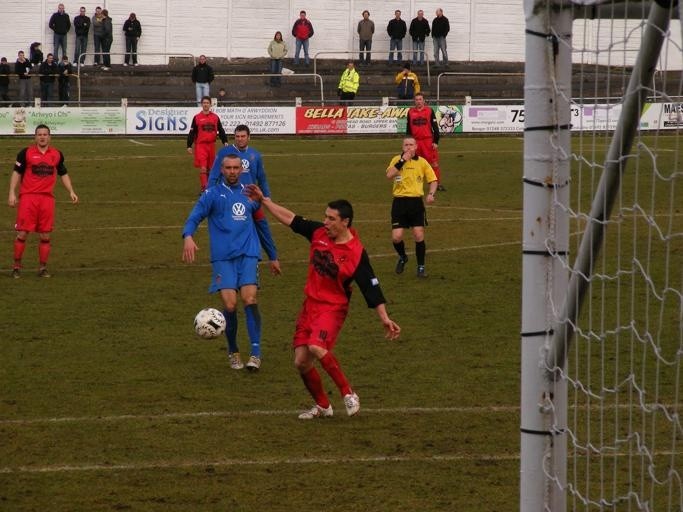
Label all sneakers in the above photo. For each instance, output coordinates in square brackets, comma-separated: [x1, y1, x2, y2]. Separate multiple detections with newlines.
[396, 256, 408, 274]
[416, 264, 425, 275]
[298, 404, 333, 421]
[343, 391, 360, 417]
[246, 355, 261, 369]
[72, 62, 111, 72]
[11, 269, 21, 279]
[38, 269, 53, 278]
[122, 63, 128, 67]
[227, 351, 243, 370]
[437, 184, 445, 192]
[133, 63, 138, 67]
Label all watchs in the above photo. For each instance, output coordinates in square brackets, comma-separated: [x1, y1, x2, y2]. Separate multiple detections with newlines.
[429, 192, 434, 197]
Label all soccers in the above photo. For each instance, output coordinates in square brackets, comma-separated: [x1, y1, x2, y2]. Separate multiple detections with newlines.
[193, 306, 227, 339]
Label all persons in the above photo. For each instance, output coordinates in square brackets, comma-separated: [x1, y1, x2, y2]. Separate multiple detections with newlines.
[191, 55, 214, 107]
[396, 64, 421, 106]
[268, 31, 288, 88]
[292, 10, 314, 67]
[182, 96, 282, 372]
[386, 8, 450, 66]
[0, 4, 142, 107]
[217, 88, 227, 107]
[386, 138, 439, 279]
[406, 93, 445, 192]
[337, 59, 360, 105]
[7, 124, 78, 279]
[242, 184, 401, 420]
[358, 10, 374, 62]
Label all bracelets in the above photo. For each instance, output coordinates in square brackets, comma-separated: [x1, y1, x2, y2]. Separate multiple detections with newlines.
[394, 158, 406, 171]
